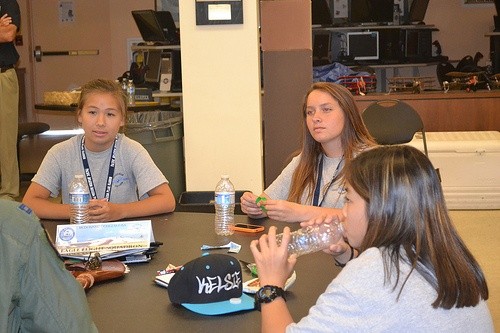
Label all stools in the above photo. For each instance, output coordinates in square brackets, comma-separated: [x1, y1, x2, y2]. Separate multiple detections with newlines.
[17, 123, 50, 195]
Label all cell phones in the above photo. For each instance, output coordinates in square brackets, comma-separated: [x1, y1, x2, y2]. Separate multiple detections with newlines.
[234, 223, 264, 233]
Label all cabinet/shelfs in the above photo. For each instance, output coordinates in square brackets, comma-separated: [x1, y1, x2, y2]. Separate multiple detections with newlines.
[312, 25, 448, 91]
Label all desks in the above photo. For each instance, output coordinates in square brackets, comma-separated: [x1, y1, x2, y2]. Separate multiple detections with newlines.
[39, 213, 343, 333]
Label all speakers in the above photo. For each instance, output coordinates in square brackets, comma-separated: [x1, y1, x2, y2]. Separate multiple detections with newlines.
[131, 10, 177, 41]
[405, 31, 432, 61]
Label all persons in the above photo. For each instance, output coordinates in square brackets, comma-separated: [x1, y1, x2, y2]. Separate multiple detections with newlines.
[250, 144, 495, 333]
[0, 0, 19, 200]
[22, 80, 176, 222]
[241, 83, 376, 223]
[0, 198, 98, 333]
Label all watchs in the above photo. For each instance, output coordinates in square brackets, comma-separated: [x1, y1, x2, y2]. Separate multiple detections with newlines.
[255, 284, 288, 312]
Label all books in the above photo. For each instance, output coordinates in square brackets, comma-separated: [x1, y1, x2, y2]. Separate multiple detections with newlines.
[151, 273, 175, 288]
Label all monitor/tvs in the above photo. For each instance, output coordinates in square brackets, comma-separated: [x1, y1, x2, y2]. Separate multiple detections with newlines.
[347, 31, 379, 59]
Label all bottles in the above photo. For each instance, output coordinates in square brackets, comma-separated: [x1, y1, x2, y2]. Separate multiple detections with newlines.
[275, 221, 346, 258]
[68, 172, 90, 224]
[126, 79, 136, 105]
[120, 78, 127, 93]
[214, 174, 235, 236]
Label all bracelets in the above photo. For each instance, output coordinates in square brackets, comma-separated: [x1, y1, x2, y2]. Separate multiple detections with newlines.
[335, 240, 355, 268]
